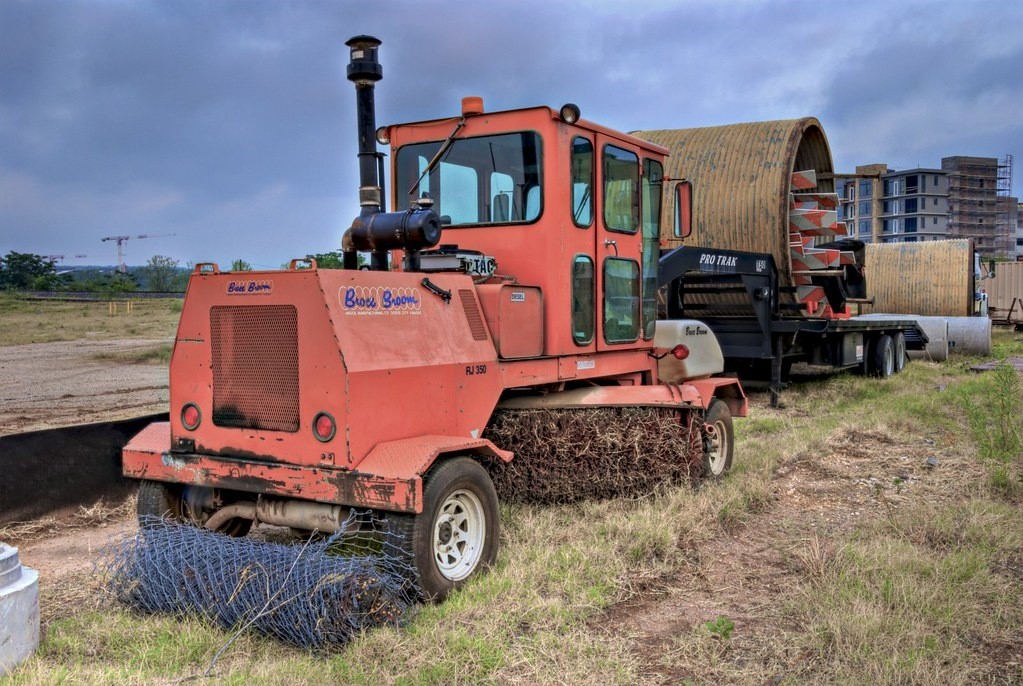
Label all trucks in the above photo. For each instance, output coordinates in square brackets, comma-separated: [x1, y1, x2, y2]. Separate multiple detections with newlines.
[973, 251, 996, 317]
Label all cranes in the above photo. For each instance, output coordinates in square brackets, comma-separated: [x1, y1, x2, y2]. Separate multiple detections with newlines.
[101, 234, 175, 274]
[43, 253, 88, 263]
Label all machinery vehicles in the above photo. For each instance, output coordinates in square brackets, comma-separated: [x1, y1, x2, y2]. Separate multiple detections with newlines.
[120, 34, 748, 608]
